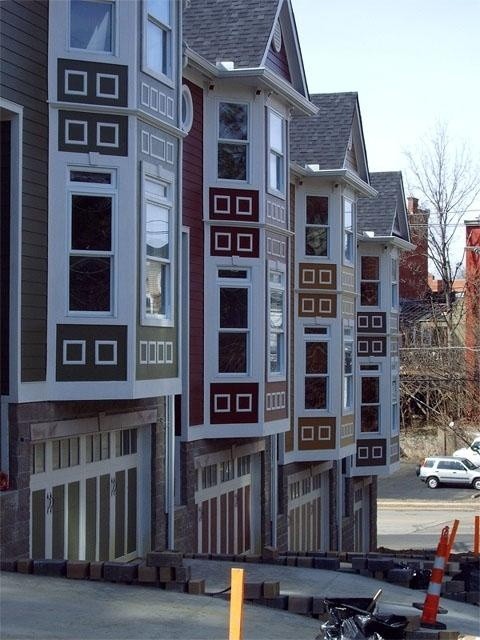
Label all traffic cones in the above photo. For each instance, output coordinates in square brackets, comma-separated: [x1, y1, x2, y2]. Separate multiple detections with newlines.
[412, 526, 450, 630]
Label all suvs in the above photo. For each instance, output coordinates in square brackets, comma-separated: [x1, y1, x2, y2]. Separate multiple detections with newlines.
[415, 456, 480, 489]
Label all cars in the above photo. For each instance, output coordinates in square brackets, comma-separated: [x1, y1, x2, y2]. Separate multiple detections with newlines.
[452, 438, 480, 467]
